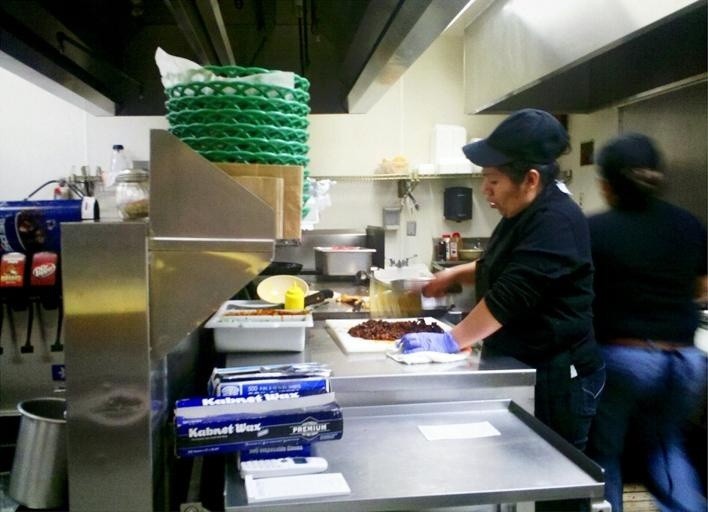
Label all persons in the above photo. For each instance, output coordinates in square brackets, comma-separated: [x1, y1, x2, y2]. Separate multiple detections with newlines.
[584, 132, 708, 512]
[395, 109, 606, 512]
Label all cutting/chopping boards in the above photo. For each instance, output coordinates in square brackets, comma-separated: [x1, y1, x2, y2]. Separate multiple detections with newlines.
[325, 314, 455, 354]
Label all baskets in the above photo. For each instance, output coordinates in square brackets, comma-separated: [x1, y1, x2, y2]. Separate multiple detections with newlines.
[160, 63, 313, 168]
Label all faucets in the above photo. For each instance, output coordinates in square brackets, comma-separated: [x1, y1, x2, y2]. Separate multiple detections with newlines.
[473, 238, 481, 248]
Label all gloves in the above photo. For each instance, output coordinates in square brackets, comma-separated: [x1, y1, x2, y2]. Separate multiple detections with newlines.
[397, 330, 461, 353]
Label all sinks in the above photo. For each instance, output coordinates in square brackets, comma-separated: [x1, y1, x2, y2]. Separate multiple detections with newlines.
[459, 248, 486, 260]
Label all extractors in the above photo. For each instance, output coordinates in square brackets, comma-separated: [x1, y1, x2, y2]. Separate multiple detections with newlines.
[1, 0, 707, 116]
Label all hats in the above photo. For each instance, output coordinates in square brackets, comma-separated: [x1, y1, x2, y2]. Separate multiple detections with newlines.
[459, 106, 570, 167]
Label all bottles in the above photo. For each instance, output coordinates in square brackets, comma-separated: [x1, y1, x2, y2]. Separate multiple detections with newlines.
[115, 172, 149, 222]
[107, 145, 133, 185]
[437, 232, 463, 261]
[283, 282, 306, 312]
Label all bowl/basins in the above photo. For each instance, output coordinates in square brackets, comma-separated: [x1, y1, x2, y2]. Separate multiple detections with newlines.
[457, 248, 482, 261]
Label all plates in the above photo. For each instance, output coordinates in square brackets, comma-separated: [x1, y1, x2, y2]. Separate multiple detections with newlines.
[257, 274, 310, 305]
